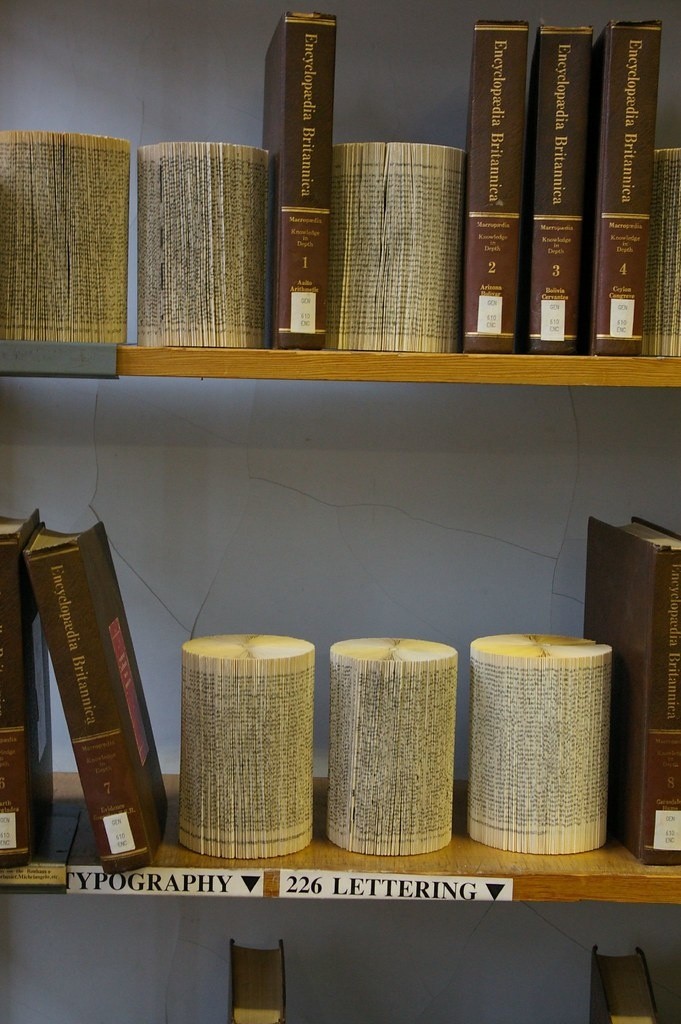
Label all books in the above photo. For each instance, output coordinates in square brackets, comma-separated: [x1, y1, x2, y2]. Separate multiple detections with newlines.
[582, 512, 681, 867]
[226, 938, 286, 1024]
[588, 945, 660, 1024]
[22, 519, 168, 876]
[259, 11, 337, 351]
[461, 18, 663, 358]
[0, 508, 53, 869]
